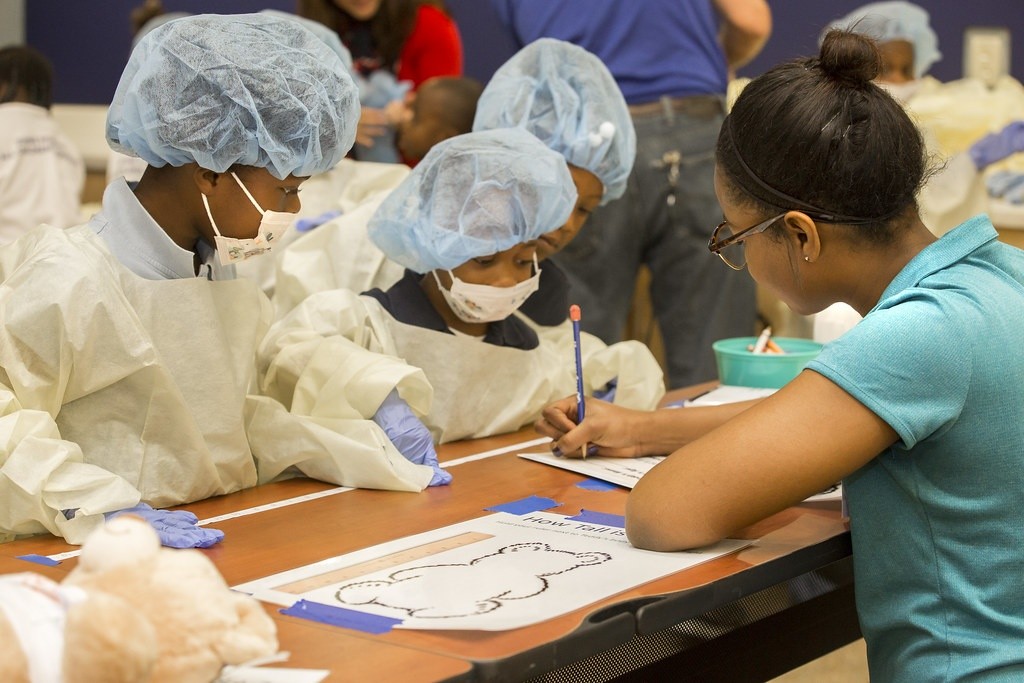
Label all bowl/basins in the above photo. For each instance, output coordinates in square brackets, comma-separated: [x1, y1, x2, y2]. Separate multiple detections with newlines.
[713, 335, 823, 386]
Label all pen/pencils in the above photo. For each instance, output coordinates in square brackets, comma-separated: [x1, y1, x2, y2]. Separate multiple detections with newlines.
[746, 324, 782, 355]
[568, 302, 588, 460]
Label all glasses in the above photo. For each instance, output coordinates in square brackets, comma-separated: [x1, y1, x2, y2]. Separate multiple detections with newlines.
[708, 211, 885, 270]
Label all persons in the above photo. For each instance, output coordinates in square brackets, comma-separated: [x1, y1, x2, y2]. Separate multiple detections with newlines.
[490, 0, 773, 393]
[820, 1, 942, 108]
[534, 14, 1023, 683]
[0, 1, 667, 550]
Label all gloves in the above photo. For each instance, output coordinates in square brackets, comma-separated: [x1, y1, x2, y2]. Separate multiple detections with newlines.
[62, 497, 224, 548]
[368, 385, 453, 486]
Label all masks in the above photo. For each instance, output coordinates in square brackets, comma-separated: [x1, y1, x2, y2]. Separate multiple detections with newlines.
[201, 169, 298, 267]
[877, 80, 919, 106]
[432, 252, 542, 323]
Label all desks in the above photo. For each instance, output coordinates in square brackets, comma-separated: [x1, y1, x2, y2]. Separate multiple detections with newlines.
[0, 380, 913, 682]
